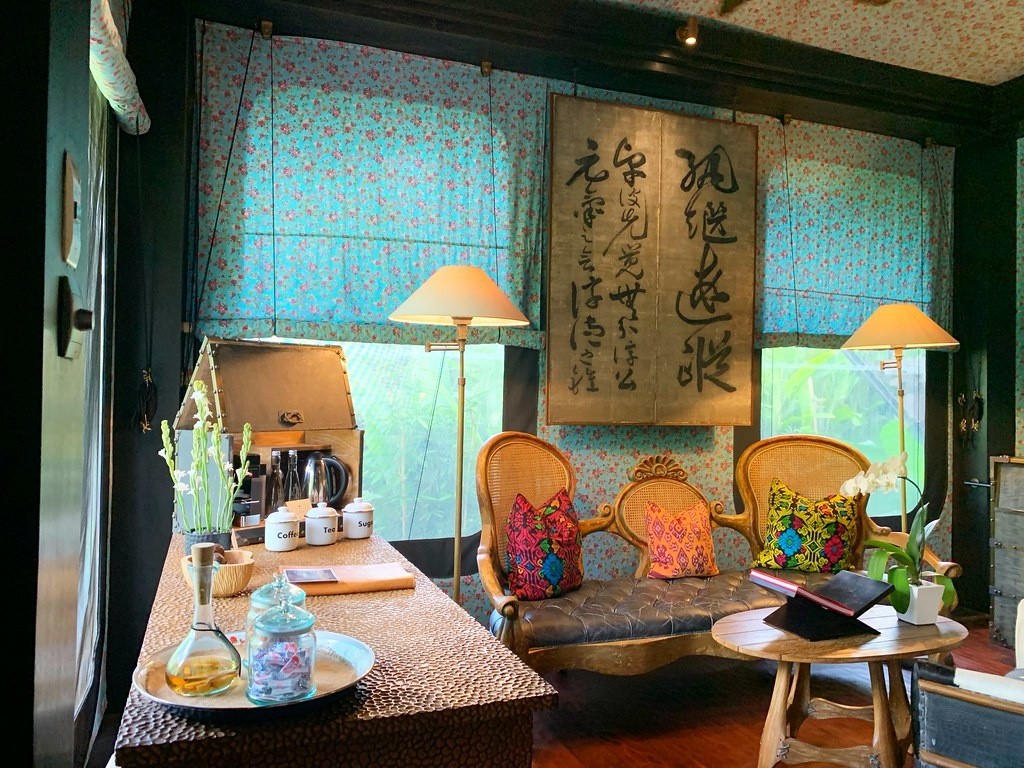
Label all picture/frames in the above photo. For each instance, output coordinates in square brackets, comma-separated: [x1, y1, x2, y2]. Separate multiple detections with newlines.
[545, 89, 758, 427]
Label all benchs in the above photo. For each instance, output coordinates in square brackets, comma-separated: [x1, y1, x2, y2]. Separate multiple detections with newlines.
[476, 430, 962, 675]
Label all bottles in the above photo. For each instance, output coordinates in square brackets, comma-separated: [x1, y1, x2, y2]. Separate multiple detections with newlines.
[166, 542, 241, 696]
[244, 572, 317, 705]
[284, 450, 309, 501]
[268, 450, 284, 512]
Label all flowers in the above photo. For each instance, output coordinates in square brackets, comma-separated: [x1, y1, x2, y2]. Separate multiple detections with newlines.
[157, 379, 253, 536]
[840, 452, 957, 613]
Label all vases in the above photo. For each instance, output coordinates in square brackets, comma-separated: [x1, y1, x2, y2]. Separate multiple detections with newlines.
[183, 527, 233, 556]
[896, 578, 945, 625]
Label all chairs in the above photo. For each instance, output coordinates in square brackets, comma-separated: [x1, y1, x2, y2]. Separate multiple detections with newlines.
[910, 598, 1024, 768]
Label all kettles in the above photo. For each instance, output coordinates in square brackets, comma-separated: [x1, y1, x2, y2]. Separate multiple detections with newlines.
[300, 451, 347, 512]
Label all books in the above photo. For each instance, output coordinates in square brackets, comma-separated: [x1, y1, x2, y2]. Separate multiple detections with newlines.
[278, 561, 416, 597]
[749, 568, 856, 617]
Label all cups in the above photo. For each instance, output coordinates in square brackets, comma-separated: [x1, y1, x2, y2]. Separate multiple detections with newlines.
[304, 502, 339, 545]
[265, 507, 299, 551]
[342, 498, 374, 539]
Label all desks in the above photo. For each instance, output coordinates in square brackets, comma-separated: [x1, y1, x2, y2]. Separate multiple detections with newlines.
[711, 605, 969, 768]
[116, 531, 558, 768]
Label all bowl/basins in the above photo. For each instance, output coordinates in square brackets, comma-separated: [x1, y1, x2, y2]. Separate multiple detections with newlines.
[182, 551, 254, 597]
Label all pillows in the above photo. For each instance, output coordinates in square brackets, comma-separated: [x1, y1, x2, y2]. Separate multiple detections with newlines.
[505, 485, 585, 600]
[754, 476, 857, 573]
[643, 498, 721, 579]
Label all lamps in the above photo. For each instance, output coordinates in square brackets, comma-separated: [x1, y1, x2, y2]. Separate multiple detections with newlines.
[388, 265, 533, 601]
[675, 16, 699, 47]
[842, 301, 961, 534]
